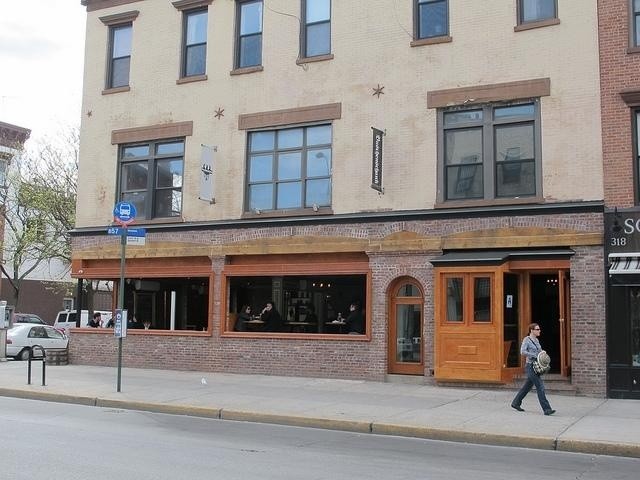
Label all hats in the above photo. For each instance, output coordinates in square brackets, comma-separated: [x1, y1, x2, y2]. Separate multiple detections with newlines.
[537, 351, 551, 368]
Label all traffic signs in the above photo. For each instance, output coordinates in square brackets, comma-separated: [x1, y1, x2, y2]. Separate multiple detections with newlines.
[113, 201, 137, 224]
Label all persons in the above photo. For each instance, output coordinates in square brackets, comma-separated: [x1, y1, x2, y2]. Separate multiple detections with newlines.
[106, 313, 115, 327]
[304, 305, 318, 333]
[127, 312, 154, 329]
[86, 313, 104, 328]
[233, 304, 255, 332]
[343, 303, 365, 335]
[511, 322, 556, 416]
[259, 301, 280, 331]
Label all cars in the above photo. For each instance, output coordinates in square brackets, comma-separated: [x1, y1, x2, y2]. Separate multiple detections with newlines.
[6, 323, 69, 362]
[53, 310, 78, 330]
[13, 312, 48, 325]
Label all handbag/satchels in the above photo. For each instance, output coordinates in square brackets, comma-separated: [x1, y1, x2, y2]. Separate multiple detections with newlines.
[533, 361, 551, 375]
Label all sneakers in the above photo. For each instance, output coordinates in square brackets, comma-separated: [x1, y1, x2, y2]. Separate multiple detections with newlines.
[544, 410, 556, 415]
[511, 403, 525, 411]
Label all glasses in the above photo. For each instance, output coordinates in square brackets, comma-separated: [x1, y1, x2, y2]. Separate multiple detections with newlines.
[532, 328, 540, 330]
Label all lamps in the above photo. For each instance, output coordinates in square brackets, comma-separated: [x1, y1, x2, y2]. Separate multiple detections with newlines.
[610, 205, 625, 234]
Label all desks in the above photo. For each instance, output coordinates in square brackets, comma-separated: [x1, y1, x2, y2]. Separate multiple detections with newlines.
[241, 319, 344, 333]
